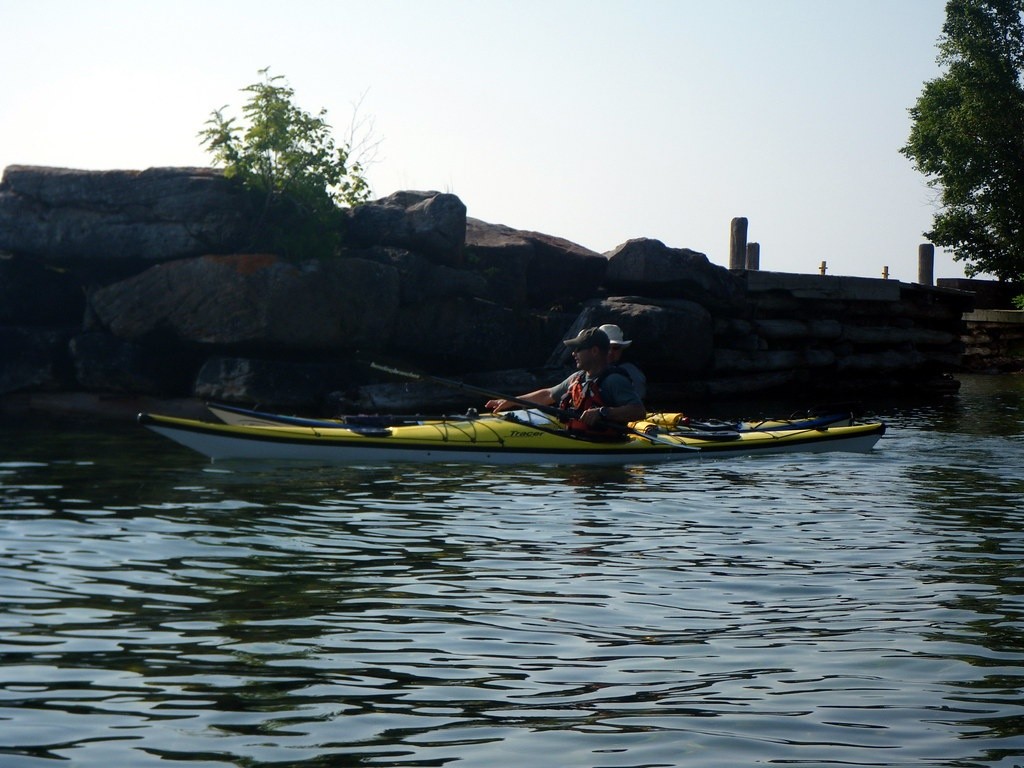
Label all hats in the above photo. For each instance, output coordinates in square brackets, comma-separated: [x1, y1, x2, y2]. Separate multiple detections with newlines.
[599, 324, 632, 348]
[563, 327, 610, 348]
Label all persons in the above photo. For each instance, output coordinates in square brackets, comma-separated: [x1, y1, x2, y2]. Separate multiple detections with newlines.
[597, 324, 647, 411]
[484, 327, 647, 442]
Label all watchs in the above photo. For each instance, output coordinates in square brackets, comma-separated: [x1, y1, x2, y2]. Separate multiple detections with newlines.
[600, 406, 610, 418]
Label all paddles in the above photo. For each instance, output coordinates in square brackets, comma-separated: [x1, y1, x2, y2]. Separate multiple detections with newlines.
[370, 359, 704, 454]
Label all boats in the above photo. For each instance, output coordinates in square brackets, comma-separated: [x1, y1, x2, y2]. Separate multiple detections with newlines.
[131, 399, 889, 467]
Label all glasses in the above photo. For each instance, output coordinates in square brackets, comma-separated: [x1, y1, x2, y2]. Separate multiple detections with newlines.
[572, 345, 591, 353]
[608, 346, 621, 351]
